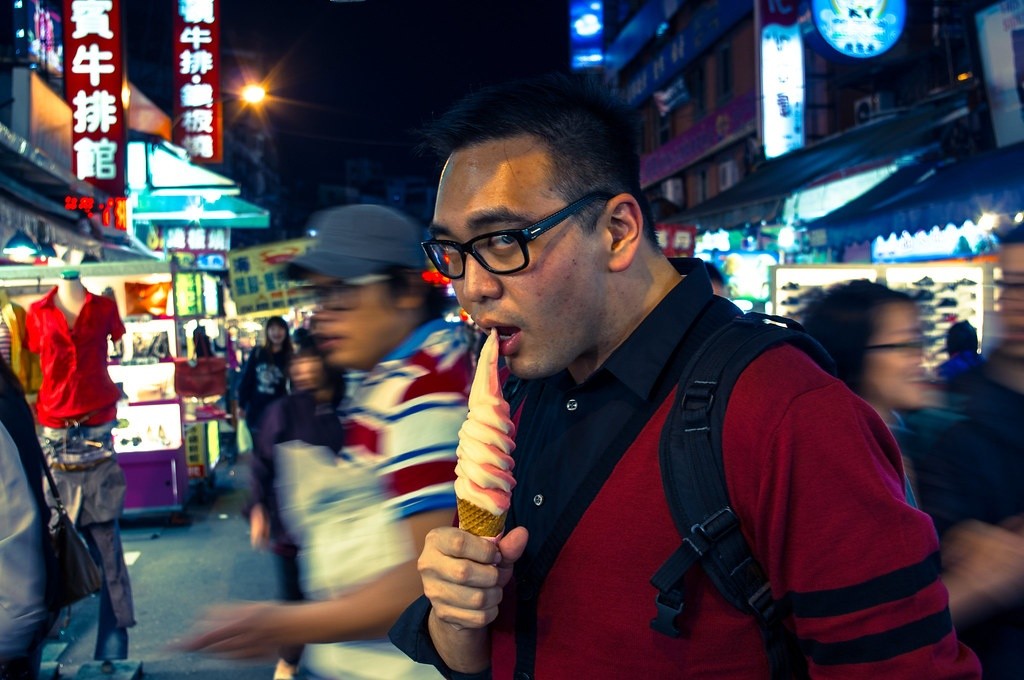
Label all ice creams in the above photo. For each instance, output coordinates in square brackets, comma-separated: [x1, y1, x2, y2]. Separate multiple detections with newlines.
[453, 331, 516, 545]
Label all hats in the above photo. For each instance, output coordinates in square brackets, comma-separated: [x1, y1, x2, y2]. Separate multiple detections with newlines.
[287, 204, 430, 277]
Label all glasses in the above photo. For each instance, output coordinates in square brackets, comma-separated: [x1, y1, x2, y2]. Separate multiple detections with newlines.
[421, 192, 616, 280]
[292, 282, 361, 310]
[865, 337, 930, 354]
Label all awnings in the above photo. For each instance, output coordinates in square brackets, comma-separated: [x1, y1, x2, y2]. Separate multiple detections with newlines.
[803, 136, 1023, 254]
[0, 123, 162, 268]
[656, 89, 984, 232]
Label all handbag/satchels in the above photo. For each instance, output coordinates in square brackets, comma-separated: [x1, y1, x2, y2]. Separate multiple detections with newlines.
[46, 515, 103, 612]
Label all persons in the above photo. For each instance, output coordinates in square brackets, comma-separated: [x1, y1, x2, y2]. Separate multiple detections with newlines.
[0, 361, 64, 680]
[0, 424, 50, 680]
[24, 266, 137, 674]
[802, 221, 1024, 679]
[162, 203, 488, 680]
[385, 90, 982, 680]
